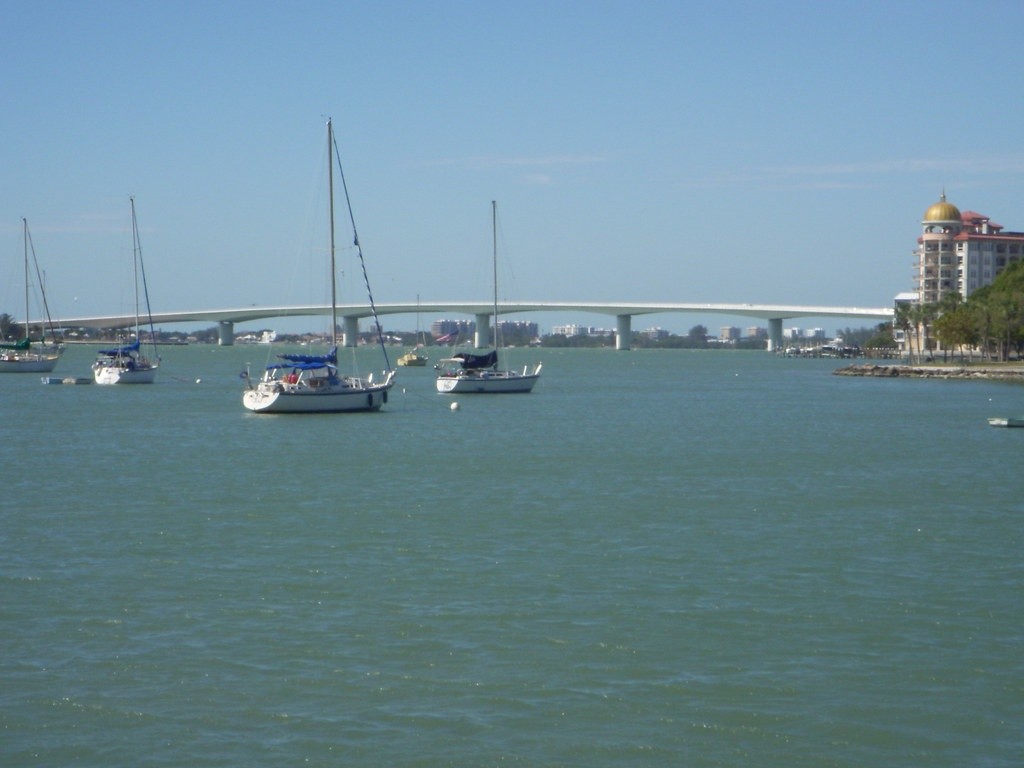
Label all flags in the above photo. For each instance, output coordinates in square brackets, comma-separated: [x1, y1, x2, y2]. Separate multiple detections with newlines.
[436, 330, 459, 344]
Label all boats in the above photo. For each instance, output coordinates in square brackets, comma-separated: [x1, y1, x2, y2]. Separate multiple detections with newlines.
[988, 415, 1024, 427]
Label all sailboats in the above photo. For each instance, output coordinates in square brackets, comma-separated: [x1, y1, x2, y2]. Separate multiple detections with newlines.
[241, 117, 398, 413]
[396, 292, 430, 367]
[0, 215, 68, 374]
[436, 201, 543, 394]
[92, 195, 164, 385]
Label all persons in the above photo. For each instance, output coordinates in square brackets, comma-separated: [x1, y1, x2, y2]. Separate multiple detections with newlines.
[447, 370, 452, 377]
[289, 370, 298, 384]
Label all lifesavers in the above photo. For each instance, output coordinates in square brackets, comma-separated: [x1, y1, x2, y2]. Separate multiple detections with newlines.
[457, 368, 465, 375]
